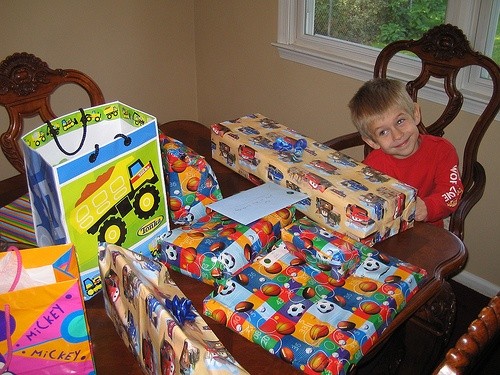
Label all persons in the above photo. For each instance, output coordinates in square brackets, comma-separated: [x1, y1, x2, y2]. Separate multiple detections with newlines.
[346, 76, 468, 227]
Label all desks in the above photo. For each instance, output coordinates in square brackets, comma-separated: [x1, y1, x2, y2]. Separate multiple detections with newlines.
[1, 119, 469, 375]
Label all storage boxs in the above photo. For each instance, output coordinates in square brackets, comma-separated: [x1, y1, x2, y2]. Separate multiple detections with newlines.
[102, 103, 428, 375]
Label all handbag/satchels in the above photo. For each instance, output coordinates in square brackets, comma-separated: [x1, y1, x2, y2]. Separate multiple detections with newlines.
[0, 244, 96, 375]
[20, 99, 170, 302]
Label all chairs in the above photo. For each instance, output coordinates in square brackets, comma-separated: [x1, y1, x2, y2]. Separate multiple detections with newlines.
[321, 24, 499, 241]
[433, 288, 500, 375]
[0, 51, 104, 174]
